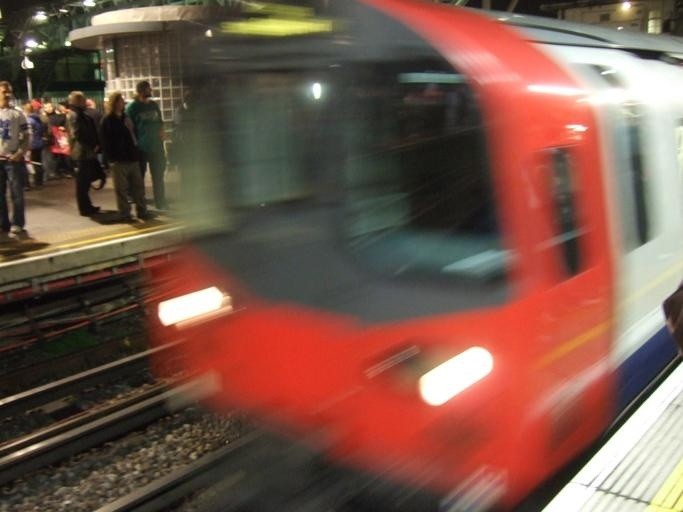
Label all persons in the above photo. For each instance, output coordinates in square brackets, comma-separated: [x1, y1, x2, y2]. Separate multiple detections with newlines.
[1, 79, 192, 244]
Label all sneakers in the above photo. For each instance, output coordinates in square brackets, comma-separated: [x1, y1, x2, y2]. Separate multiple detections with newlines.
[11, 225, 22, 234]
[81, 205, 100, 216]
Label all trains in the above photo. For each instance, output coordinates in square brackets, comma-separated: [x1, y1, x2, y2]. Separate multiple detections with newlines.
[144, 0, 681, 511]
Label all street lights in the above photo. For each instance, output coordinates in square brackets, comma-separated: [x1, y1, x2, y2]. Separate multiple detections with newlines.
[20, 37, 39, 100]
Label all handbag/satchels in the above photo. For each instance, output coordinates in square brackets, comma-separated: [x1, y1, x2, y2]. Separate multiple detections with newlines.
[91, 157, 103, 181]
